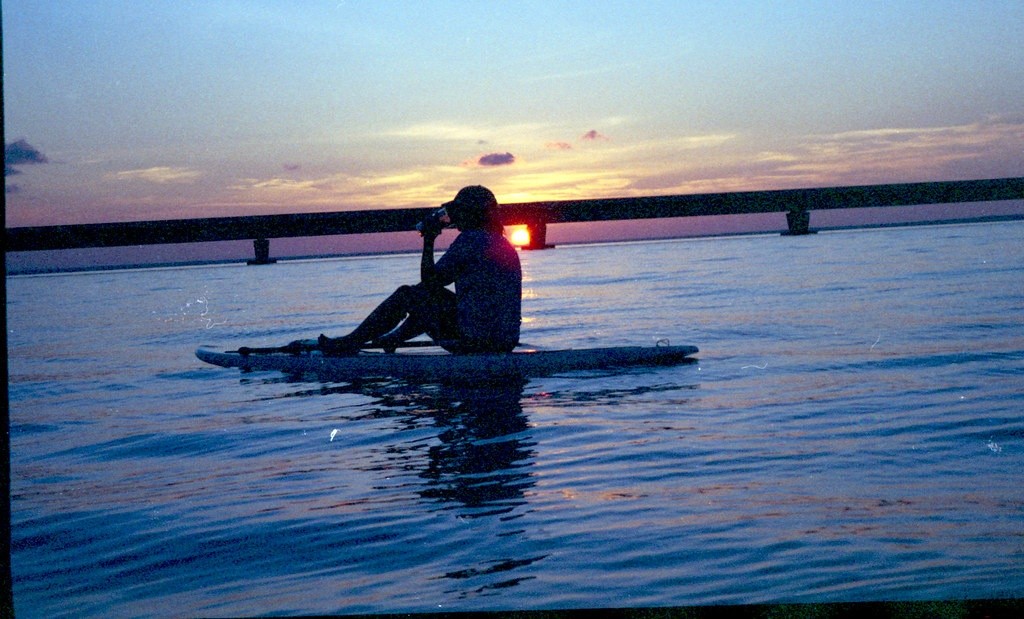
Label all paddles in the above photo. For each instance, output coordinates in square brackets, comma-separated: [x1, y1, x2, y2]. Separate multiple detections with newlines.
[227, 338, 573, 352]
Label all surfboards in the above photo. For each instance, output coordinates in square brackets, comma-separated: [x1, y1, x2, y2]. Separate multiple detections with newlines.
[195, 345, 699, 380]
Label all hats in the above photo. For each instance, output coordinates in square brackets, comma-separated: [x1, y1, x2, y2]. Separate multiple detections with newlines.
[441, 185, 501, 212]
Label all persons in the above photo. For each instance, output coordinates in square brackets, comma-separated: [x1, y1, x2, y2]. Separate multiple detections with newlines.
[317, 186, 522, 354]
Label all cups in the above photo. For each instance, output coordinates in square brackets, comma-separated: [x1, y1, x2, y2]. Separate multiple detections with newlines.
[415, 205, 453, 237]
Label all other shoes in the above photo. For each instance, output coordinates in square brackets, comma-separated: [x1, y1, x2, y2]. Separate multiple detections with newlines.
[372, 337, 400, 354]
[318, 334, 360, 357]
